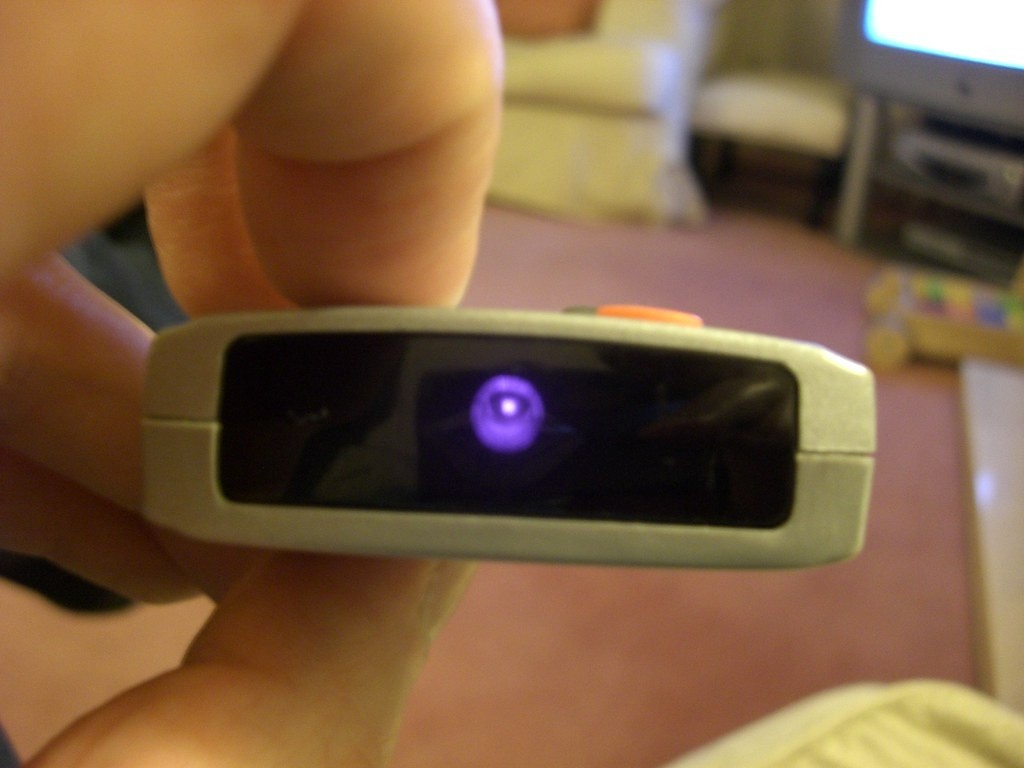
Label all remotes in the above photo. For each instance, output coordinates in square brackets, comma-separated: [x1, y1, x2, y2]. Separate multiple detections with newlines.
[147, 301, 877, 574]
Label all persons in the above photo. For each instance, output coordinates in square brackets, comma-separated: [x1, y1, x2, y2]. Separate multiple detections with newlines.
[1, 0, 511, 762]
